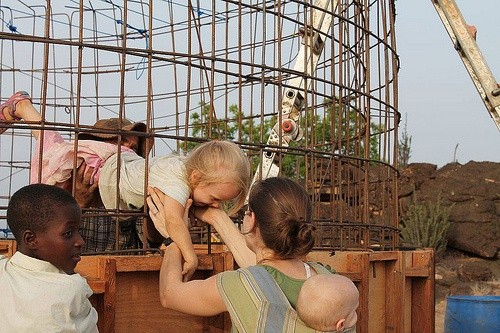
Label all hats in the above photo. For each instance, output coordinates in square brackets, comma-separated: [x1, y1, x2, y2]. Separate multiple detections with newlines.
[78, 118, 154, 158]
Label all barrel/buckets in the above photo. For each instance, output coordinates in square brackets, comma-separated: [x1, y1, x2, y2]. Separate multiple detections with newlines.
[444, 295, 500, 333]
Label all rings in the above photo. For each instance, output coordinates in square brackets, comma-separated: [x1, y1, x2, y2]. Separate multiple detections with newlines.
[154, 211, 158, 216]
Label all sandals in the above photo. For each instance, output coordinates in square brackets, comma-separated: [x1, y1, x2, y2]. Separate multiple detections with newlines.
[0, 91, 32, 134]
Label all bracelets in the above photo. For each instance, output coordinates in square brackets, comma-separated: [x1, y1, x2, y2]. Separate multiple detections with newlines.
[164, 237, 174, 245]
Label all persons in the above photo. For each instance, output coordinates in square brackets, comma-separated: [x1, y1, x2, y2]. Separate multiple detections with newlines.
[55, 118, 154, 253]
[0, 183, 98, 333]
[1, 91, 248, 283]
[147, 176, 357, 333]
[296, 273, 359, 333]
